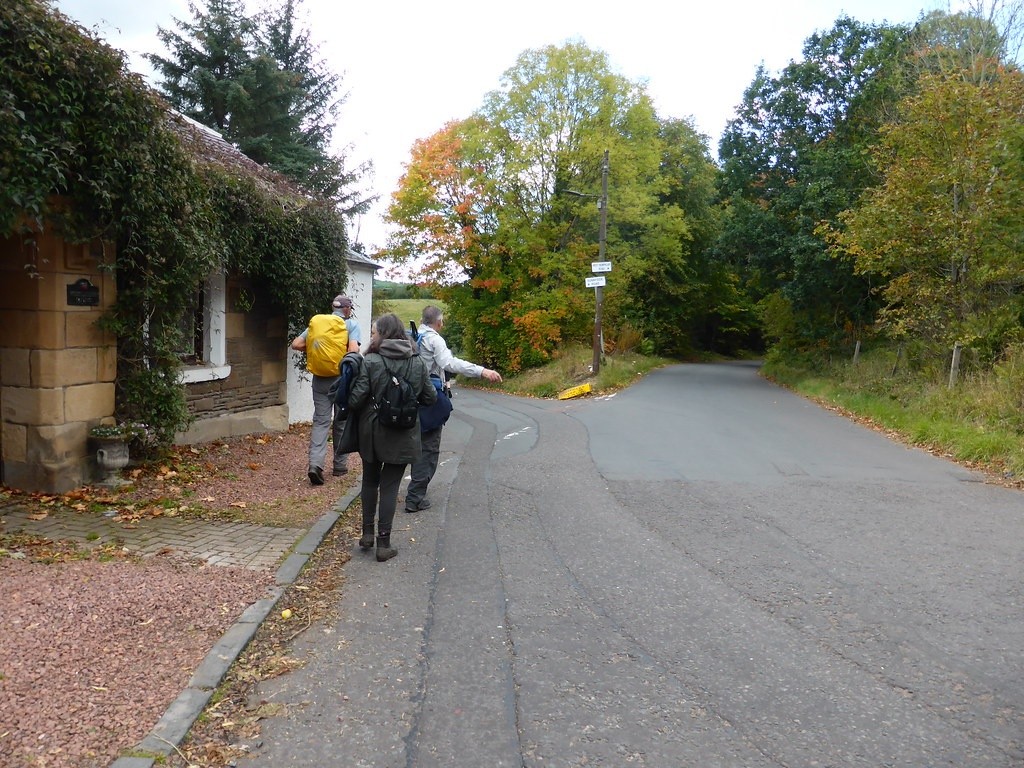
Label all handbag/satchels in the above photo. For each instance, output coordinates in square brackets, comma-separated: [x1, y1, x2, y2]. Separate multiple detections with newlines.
[419, 375, 453, 432]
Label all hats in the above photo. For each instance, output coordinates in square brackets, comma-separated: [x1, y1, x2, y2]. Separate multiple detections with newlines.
[333, 294, 355, 310]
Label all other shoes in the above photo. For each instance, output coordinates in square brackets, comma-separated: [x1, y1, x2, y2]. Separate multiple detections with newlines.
[332, 467, 348, 476]
[308, 466, 324, 485]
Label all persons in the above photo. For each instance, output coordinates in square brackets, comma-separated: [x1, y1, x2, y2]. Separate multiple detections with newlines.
[291, 295, 362, 484]
[405, 305, 503, 562]
[340, 313, 438, 563]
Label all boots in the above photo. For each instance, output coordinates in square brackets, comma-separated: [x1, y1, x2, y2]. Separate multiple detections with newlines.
[376, 535, 397, 562]
[359, 523, 375, 551]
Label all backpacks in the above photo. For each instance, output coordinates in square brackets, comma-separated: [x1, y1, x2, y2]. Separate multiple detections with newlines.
[368, 353, 419, 429]
[306, 313, 350, 377]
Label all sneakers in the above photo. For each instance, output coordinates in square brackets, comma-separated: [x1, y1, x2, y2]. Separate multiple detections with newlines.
[406, 499, 431, 512]
